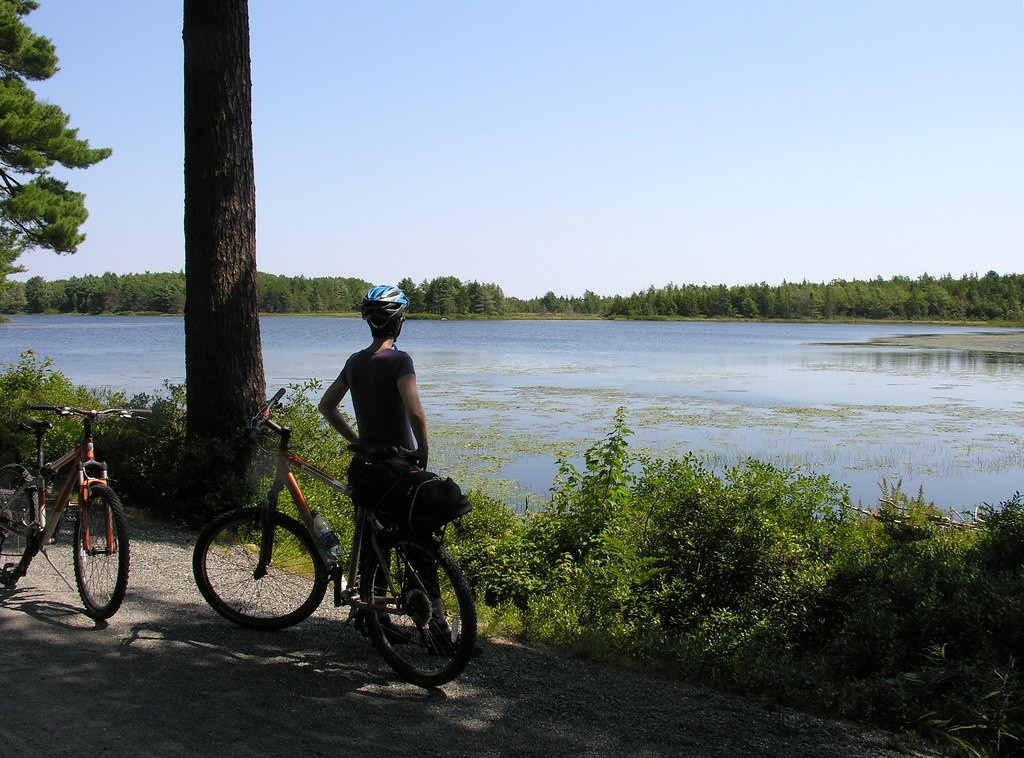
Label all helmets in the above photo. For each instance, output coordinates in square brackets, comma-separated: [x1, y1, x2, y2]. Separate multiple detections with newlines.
[360, 285, 410, 320]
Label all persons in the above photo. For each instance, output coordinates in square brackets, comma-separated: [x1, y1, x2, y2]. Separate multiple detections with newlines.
[317, 284, 483, 656]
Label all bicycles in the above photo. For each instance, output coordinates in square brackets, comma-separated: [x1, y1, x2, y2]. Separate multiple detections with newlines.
[193, 388, 477, 688]
[0, 405, 152, 619]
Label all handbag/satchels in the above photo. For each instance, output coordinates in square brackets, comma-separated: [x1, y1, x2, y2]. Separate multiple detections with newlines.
[372, 455, 471, 536]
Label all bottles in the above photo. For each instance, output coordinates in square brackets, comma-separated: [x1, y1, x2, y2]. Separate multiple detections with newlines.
[311, 510, 344, 557]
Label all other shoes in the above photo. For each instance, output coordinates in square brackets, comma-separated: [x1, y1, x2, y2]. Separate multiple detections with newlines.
[362, 621, 411, 645]
[430, 636, 482, 659]
